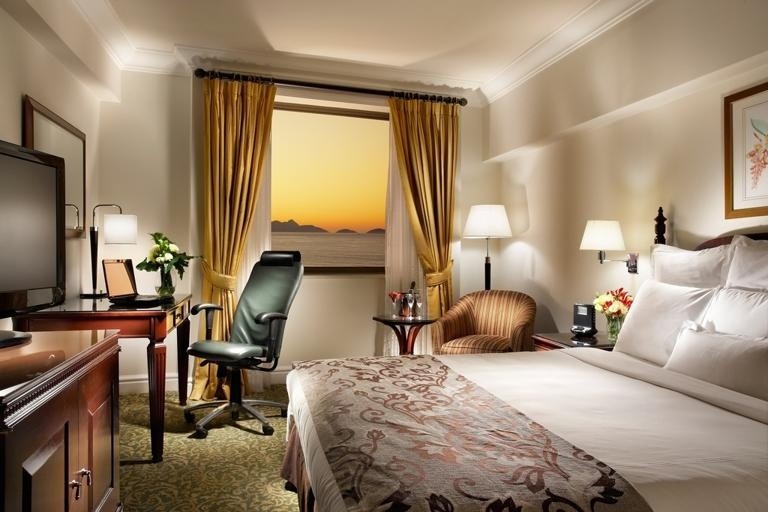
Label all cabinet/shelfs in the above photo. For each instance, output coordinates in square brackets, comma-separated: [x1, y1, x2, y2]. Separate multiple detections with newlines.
[0, 328, 122, 512]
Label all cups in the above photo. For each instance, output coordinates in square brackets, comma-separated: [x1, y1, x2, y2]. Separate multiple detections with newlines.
[407, 294, 415, 321]
[414, 294, 424, 320]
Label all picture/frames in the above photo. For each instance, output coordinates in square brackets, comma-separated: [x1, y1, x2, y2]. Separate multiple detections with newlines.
[722, 77, 768, 219]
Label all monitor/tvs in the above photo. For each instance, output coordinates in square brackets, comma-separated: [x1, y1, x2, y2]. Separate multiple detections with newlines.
[1, 141, 66, 349]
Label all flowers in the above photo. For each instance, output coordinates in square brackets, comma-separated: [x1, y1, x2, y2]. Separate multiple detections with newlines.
[136, 231, 193, 280]
[593, 288, 633, 317]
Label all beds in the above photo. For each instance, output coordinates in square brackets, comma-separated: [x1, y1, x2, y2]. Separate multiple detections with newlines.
[286, 224, 768, 512]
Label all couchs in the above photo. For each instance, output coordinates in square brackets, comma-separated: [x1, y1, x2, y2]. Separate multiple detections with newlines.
[433, 288, 538, 356]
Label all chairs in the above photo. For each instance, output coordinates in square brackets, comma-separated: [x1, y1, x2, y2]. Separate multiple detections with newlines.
[180, 248, 305, 439]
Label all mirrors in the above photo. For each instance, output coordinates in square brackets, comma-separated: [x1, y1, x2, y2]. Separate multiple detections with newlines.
[22, 92, 88, 242]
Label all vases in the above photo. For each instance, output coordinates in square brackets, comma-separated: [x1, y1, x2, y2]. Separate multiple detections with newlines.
[606, 314, 623, 340]
[156, 262, 177, 297]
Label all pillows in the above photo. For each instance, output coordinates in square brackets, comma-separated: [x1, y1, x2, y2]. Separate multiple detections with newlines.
[723, 232, 768, 292]
[697, 286, 768, 341]
[651, 242, 729, 288]
[613, 279, 718, 367]
[665, 321, 768, 402]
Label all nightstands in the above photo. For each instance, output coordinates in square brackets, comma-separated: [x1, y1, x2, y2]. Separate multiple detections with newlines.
[528, 330, 616, 353]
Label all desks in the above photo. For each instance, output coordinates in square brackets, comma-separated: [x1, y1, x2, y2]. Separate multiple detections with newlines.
[11, 288, 192, 463]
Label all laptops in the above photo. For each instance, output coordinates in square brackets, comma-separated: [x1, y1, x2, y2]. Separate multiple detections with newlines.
[102, 259, 174, 305]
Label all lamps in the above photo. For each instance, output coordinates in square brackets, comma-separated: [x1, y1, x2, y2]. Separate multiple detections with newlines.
[461, 204, 514, 290]
[577, 219, 638, 275]
[79, 202, 139, 299]
